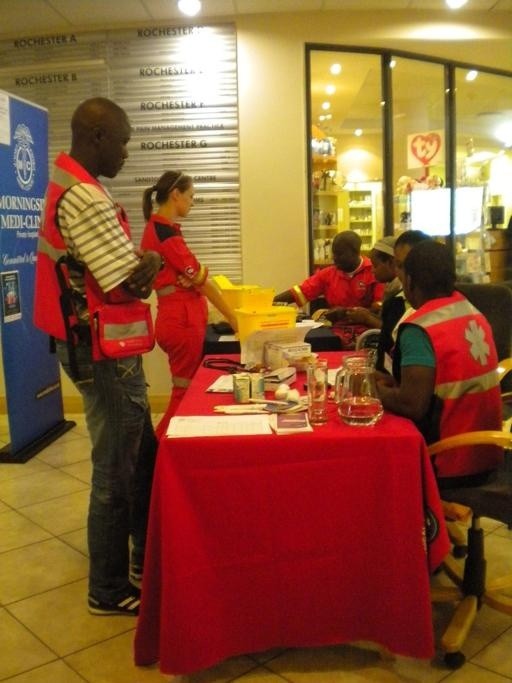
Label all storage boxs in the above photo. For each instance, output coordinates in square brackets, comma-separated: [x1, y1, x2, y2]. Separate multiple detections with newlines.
[233, 306, 298, 350]
[212, 275, 276, 309]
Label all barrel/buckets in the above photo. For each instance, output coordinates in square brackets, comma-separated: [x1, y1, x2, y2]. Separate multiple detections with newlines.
[234, 305, 299, 364]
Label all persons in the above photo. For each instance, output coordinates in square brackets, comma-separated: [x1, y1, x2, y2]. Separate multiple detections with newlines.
[141, 167, 239, 444]
[375, 238, 503, 485]
[272, 227, 386, 350]
[325, 232, 405, 329]
[31, 94, 168, 617]
[373, 227, 432, 376]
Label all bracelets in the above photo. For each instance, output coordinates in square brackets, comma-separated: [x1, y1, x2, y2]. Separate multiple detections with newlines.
[160, 253, 165, 273]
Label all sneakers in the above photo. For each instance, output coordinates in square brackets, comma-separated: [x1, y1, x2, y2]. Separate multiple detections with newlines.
[87, 582, 141, 616]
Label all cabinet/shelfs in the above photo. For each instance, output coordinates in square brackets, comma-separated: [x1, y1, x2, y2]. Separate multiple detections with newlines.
[349, 182, 385, 251]
[312, 191, 350, 268]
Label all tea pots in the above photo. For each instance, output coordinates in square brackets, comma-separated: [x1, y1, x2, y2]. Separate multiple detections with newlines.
[332, 352, 384, 426]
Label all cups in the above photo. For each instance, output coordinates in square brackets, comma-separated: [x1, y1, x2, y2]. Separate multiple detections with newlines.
[307, 364, 328, 426]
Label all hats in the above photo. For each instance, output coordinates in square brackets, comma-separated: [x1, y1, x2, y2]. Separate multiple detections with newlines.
[373, 236, 398, 257]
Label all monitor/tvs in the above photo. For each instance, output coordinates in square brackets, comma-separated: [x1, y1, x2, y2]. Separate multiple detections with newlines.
[486, 206, 504, 228]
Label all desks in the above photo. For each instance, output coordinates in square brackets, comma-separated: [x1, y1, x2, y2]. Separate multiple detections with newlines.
[133, 326, 453, 676]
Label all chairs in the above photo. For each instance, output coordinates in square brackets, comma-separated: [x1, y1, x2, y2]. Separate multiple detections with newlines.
[427, 417, 512, 669]
[454, 283, 512, 403]
